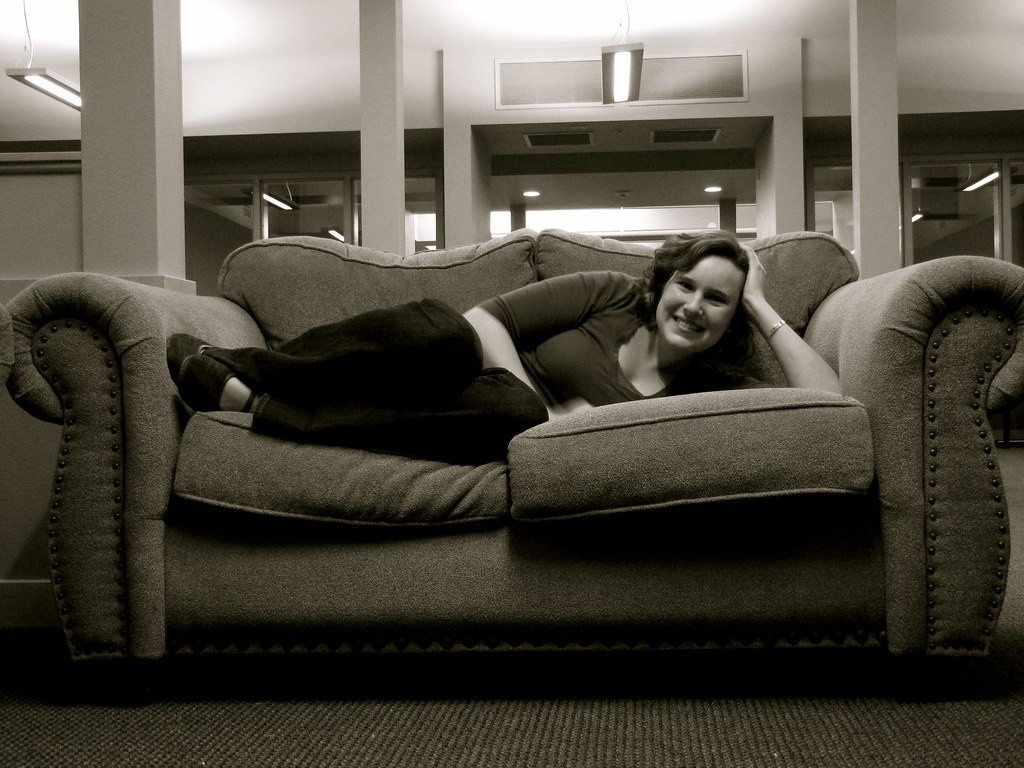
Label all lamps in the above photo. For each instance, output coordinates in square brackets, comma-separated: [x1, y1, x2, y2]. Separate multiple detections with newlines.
[601, 0, 644, 105]
[912, 210, 925, 223]
[322, 226, 344, 242]
[5, 0, 81, 112]
[954, 163, 1018, 192]
[240, 182, 300, 210]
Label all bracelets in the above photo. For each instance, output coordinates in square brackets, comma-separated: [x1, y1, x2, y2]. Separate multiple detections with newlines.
[766, 320, 785, 337]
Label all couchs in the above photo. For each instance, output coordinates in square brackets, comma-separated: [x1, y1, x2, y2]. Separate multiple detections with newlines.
[0, 227, 1024, 664]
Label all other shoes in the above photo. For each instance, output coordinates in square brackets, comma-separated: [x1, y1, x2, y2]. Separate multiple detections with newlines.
[177, 354, 257, 413]
[166, 332, 214, 386]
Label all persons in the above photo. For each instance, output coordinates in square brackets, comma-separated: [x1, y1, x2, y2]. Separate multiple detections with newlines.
[166, 227, 840, 465]
